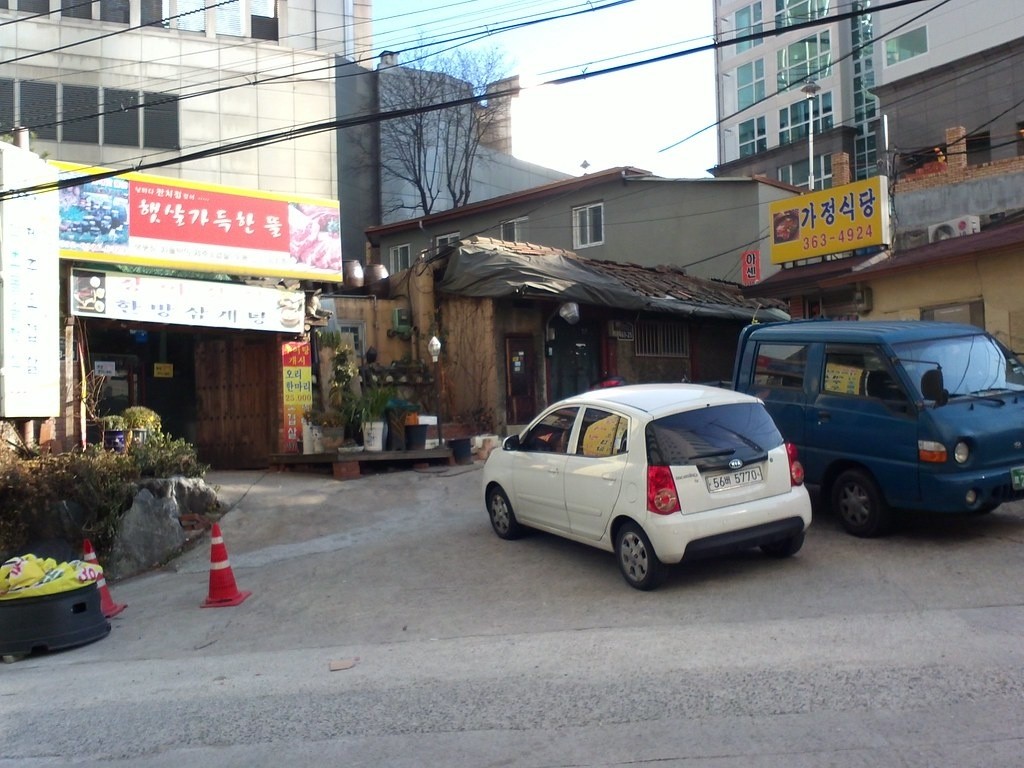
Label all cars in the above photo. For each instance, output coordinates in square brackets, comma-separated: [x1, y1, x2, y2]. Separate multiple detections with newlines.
[481, 383, 812, 591]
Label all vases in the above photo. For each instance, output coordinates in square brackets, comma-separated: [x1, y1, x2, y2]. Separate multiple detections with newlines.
[405, 424, 427, 449]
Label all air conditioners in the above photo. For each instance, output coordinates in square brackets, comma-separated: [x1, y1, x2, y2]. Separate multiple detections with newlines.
[925, 215, 980, 242]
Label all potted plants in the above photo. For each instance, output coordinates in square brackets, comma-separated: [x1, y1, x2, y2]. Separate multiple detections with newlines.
[345, 380, 387, 451]
[318, 414, 344, 452]
[100, 414, 130, 451]
[300, 411, 313, 454]
[124, 407, 163, 448]
[310, 413, 324, 453]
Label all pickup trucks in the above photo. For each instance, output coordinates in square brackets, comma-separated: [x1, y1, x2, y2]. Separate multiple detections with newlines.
[700, 317, 1024, 537]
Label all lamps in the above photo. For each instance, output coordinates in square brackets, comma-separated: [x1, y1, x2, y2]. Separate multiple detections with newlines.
[800, 77, 821, 100]
[557, 301, 582, 325]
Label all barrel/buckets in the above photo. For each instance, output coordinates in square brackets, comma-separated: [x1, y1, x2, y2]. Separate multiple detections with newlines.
[405, 424, 428, 451]
[447, 439, 475, 466]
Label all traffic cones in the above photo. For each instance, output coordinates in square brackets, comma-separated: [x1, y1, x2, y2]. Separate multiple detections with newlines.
[199, 522, 252, 608]
[83, 538, 128, 619]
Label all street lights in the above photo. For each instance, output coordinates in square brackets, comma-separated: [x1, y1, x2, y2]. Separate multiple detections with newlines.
[428, 336, 449, 450]
[800, 81, 821, 191]
